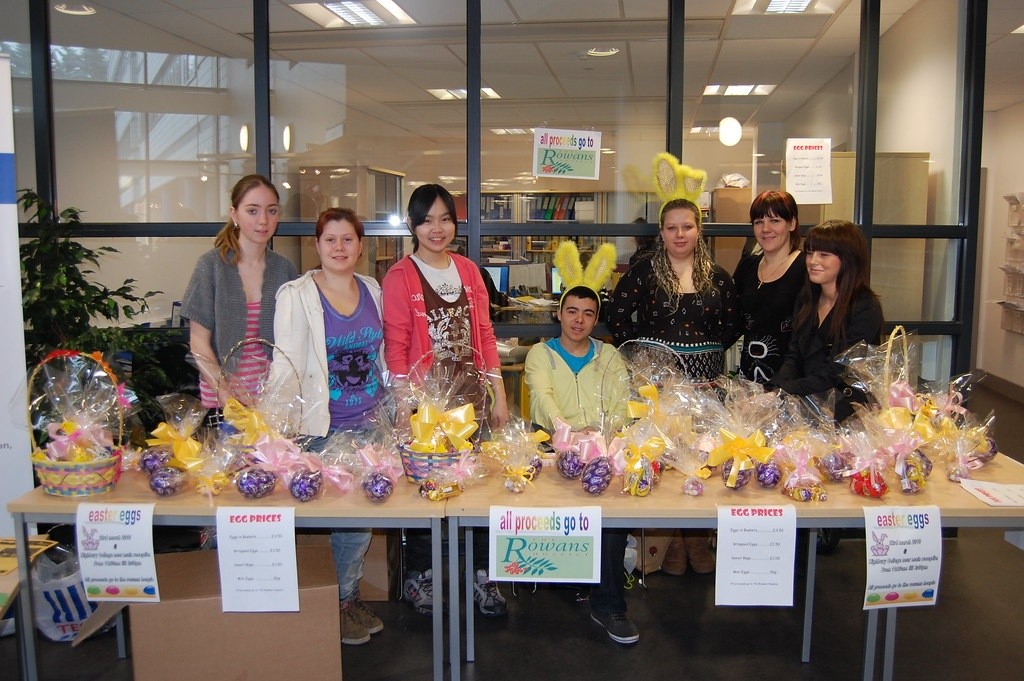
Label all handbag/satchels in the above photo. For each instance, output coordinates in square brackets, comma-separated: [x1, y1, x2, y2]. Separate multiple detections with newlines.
[29, 566, 118, 641]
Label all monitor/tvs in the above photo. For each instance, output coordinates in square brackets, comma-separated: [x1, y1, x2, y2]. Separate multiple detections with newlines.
[551, 265, 563, 295]
[480, 265, 510, 295]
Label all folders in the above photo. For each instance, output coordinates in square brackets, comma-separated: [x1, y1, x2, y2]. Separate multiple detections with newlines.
[564, 196, 575, 220]
[552, 196, 564, 220]
[540, 195, 551, 219]
[557, 197, 570, 220]
[536, 195, 545, 219]
[527, 236, 594, 264]
[530, 196, 536, 219]
[171, 300, 184, 327]
[481, 196, 511, 219]
[570, 196, 581, 220]
[114, 351, 133, 365]
[581, 196, 592, 201]
[545, 196, 557, 220]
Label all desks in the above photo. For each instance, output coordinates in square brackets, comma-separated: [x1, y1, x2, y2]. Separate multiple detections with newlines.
[445, 445, 1024, 681]
[490, 304, 558, 317]
[7, 454, 463, 681]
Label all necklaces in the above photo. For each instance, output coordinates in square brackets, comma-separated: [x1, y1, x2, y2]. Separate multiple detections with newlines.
[417, 251, 450, 269]
[757, 255, 789, 289]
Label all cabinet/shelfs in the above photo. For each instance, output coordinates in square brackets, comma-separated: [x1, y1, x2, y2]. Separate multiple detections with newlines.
[301, 150, 929, 390]
[999, 193, 1024, 335]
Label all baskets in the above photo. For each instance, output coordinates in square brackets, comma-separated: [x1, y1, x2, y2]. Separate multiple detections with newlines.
[28, 350, 123, 496]
[393, 343, 487, 485]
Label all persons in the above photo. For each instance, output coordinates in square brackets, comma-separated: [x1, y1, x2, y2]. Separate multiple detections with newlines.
[629, 217, 656, 269]
[480, 268, 508, 323]
[762, 219, 886, 559]
[721, 189, 806, 390]
[179, 174, 299, 428]
[258, 207, 389, 644]
[602, 198, 734, 576]
[381, 185, 508, 618]
[524, 285, 639, 644]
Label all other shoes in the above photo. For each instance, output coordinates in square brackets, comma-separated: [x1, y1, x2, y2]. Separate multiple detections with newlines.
[816, 527, 841, 553]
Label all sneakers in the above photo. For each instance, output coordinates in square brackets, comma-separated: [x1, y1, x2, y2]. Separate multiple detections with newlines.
[472, 569, 508, 617]
[684, 537, 713, 574]
[590, 608, 640, 643]
[403, 568, 447, 616]
[348, 597, 383, 634]
[661, 537, 687, 577]
[341, 608, 371, 644]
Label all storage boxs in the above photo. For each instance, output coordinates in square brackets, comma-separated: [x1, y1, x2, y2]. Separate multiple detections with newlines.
[72, 529, 391, 681]
